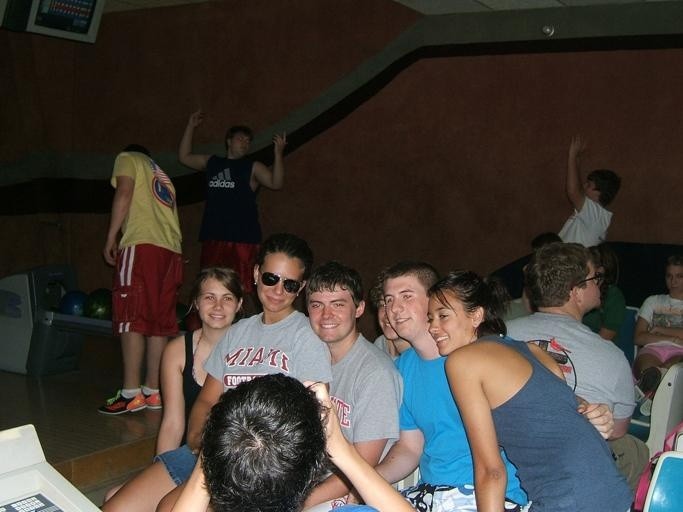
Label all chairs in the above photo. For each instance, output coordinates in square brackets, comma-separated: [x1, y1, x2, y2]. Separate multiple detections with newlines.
[617, 361, 683, 465]
[615, 305, 639, 371]
[640, 450, 683, 512]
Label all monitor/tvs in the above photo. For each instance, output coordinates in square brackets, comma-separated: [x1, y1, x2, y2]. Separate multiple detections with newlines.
[0, 0, 105, 44]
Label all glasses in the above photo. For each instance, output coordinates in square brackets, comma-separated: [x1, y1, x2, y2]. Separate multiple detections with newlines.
[255, 268, 304, 295]
[572, 272, 604, 288]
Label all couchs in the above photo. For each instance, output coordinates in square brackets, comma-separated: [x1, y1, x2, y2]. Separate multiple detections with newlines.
[488, 236, 682, 310]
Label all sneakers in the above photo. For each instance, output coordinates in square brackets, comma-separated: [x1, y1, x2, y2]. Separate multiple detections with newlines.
[96, 389, 163, 416]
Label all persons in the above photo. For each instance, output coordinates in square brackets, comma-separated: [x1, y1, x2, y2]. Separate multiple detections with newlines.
[178, 106, 289, 317]
[99, 137, 187, 416]
[558, 133, 622, 248]
[100, 231, 682, 512]
